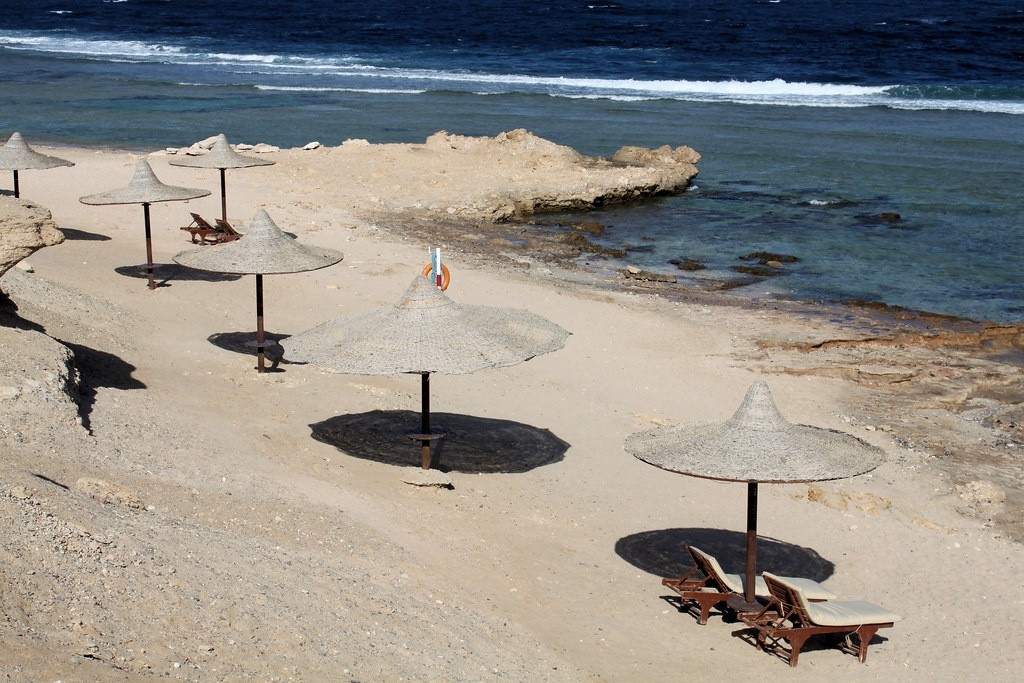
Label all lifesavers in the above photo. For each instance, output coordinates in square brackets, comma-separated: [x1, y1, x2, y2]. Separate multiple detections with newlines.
[422, 263, 451, 292]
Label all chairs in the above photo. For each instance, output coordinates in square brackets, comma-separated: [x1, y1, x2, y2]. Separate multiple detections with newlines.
[180, 212, 225, 246]
[737, 571, 901, 667]
[662, 544, 837, 625]
[206, 218, 244, 244]
